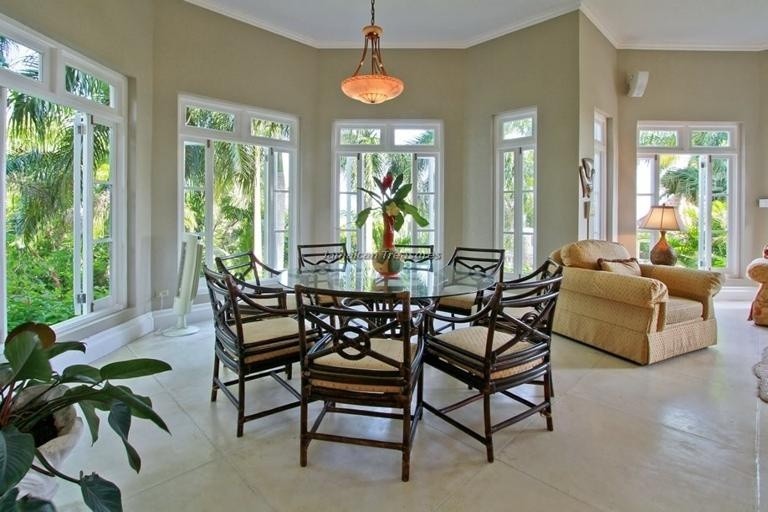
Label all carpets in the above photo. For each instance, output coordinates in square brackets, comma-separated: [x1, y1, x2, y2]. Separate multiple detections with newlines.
[751, 346, 768, 403]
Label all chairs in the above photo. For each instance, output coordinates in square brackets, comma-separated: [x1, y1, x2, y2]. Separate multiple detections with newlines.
[201, 261, 327, 438]
[296, 243, 368, 329]
[747, 245, 768, 326]
[214, 249, 296, 373]
[547, 248, 726, 367]
[393, 244, 434, 298]
[296, 284, 424, 482]
[433, 246, 505, 332]
[422, 275, 563, 462]
[468, 259, 562, 396]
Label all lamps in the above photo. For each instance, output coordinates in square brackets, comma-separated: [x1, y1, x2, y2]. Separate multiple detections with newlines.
[639, 203, 682, 265]
[340, 0, 404, 106]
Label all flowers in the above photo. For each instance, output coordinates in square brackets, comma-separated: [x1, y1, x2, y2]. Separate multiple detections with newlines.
[353, 172, 430, 232]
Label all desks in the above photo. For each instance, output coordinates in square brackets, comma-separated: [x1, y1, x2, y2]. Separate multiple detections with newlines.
[276, 261, 496, 341]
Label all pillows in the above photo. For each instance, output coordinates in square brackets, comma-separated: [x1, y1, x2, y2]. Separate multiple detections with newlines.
[560, 238, 631, 270]
[596, 257, 642, 276]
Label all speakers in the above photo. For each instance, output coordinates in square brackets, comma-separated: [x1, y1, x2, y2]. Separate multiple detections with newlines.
[626, 71, 650, 98]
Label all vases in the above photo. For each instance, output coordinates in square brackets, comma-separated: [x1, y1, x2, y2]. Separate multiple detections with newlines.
[371, 212, 404, 276]
